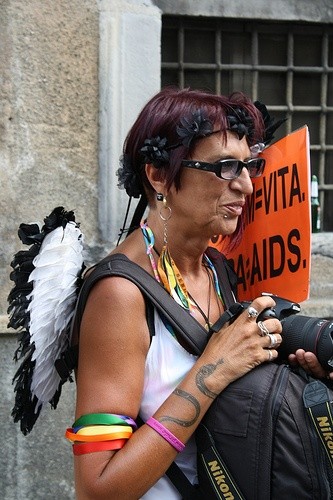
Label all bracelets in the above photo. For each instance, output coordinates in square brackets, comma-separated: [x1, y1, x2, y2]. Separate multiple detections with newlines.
[146, 417, 185, 452]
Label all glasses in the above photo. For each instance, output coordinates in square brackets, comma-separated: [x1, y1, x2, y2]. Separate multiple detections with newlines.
[181, 158, 266, 181]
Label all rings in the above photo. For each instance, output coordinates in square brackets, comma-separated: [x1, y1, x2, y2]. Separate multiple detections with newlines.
[268, 350, 272, 360]
[247, 306, 259, 317]
[258, 321, 269, 334]
[266, 334, 277, 348]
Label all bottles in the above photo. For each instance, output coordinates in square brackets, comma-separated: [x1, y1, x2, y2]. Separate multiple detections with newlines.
[310, 175, 321, 233]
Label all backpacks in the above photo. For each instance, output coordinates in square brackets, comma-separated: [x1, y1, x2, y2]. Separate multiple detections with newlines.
[66, 246, 333, 500]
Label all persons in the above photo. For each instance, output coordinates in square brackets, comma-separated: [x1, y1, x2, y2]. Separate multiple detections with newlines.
[65, 87, 333, 500]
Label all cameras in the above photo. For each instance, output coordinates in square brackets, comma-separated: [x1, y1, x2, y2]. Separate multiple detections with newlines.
[230, 292, 333, 374]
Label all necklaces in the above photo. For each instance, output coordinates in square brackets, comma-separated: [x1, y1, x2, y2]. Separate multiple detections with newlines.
[154, 248, 214, 332]
